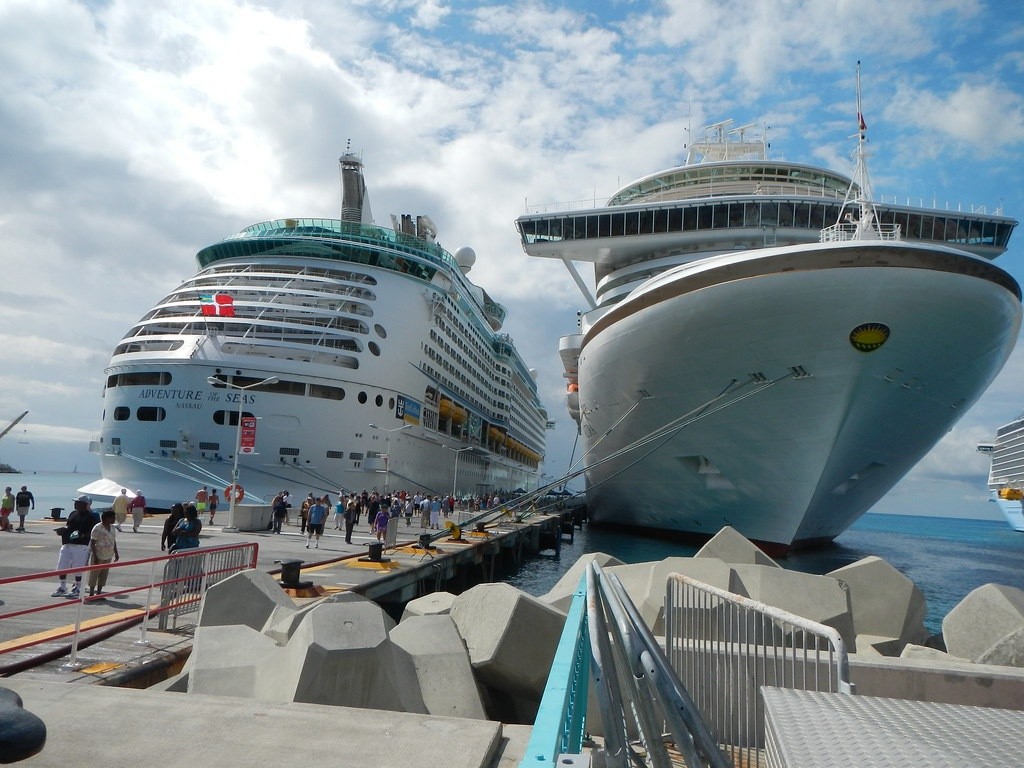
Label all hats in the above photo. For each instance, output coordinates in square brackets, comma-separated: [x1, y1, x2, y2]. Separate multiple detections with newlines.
[307, 497, 312, 500]
[71, 494, 90, 504]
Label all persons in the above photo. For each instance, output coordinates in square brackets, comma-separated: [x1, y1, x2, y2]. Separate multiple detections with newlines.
[195, 485, 209, 516]
[51, 494, 101, 599]
[130, 490, 146, 533]
[361, 488, 455, 535]
[16, 486, 34, 531]
[373, 504, 391, 544]
[272, 489, 293, 534]
[458, 491, 529, 512]
[0, 487, 15, 531]
[159, 498, 204, 583]
[333, 488, 362, 544]
[208, 489, 220, 525]
[90, 510, 119, 600]
[113, 489, 131, 532]
[299, 490, 332, 549]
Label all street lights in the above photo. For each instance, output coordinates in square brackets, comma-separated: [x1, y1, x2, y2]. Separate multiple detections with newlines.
[442, 445, 473, 501]
[207, 375, 279, 533]
[369, 424, 413, 498]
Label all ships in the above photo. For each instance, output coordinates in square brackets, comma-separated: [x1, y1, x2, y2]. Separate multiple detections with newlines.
[73, 139, 556, 511]
[976, 413, 1024, 531]
[514, 60, 1023, 558]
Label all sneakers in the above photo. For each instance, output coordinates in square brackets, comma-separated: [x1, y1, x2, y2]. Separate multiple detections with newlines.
[66, 587, 80, 599]
[51, 587, 69, 597]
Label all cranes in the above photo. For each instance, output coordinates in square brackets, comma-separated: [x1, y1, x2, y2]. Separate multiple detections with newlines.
[0, 409, 31, 444]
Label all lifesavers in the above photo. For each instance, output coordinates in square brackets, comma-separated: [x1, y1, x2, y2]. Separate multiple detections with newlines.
[225, 484, 244, 502]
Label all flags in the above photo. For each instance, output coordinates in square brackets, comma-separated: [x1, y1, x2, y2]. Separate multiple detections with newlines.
[856, 112, 868, 130]
[198, 294, 236, 318]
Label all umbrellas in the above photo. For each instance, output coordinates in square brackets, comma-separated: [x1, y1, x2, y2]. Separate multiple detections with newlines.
[513, 487, 527, 495]
[546, 490, 558, 496]
[559, 490, 572, 498]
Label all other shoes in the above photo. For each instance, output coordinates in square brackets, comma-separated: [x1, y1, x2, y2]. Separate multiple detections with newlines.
[0, 524, 13, 531]
[346, 540, 352, 544]
[339, 528, 341, 530]
[314, 545, 318, 548]
[300, 531, 304, 534]
[115, 525, 122, 532]
[334, 526, 338, 530]
[209, 520, 213, 525]
[132, 526, 137, 533]
[16, 527, 25, 533]
[306, 544, 309, 549]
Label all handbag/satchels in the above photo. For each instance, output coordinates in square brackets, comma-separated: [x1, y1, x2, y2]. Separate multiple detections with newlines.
[268, 520, 274, 529]
[168, 543, 176, 554]
